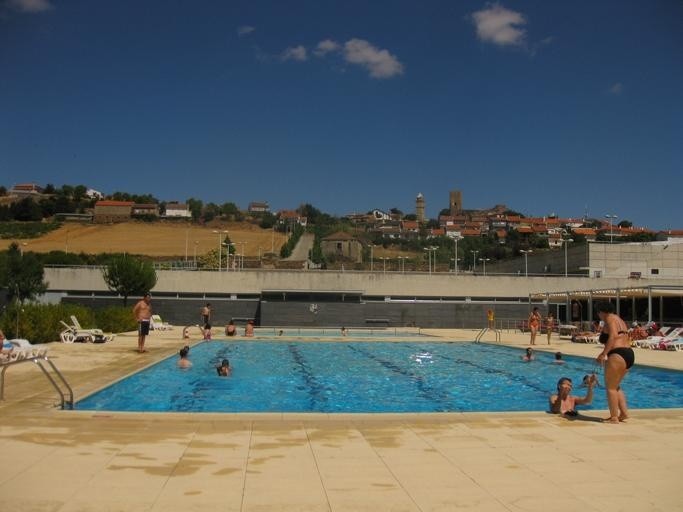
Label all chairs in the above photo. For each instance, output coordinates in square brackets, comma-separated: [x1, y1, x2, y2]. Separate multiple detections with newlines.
[59, 321, 105, 343]
[69, 315, 116, 341]
[573, 320, 682, 350]
[151, 314, 172, 336]
[148, 317, 166, 334]
[0, 338, 50, 368]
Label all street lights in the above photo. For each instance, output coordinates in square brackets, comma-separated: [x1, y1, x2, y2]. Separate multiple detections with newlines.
[19, 242, 27, 256]
[469, 250, 479, 271]
[380, 257, 388, 274]
[63, 228, 69, 256]
[604, 214, 618, 243]
[367, 243, 376, 272]
[258, 246, 262, 261]
[519, 249, 534, 276]
[397, 255, 408, 274]
[449, 234, 462, 275]
[479, 257, 491, 276]
[557, 238, 574, 277]
[180, 222, 247, 272]
[423, 245, 440, 276]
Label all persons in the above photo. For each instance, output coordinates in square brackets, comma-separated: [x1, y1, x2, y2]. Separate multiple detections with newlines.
[552, 351, 564, 362]
[223, 320, 237, 336]
[593, 300, 634, 422]
[216, 360, 232, 376]
[133, 293, 153, 353]
[339, 326, 351, 337]
[183, 346, 191, 355]
[199, 324, 210, 340]
[544, 312, 554, 345]
[528, 305, 541, 345]
[521, 348, 535, 361]
[0, 330, 13, 355]
[276, 331, 283, 336]
[200, 303, 211, 324]
[176, 349, 191, 367]
[548, 375, 596, 413]
[574, 320, 663, 346]
[245, 320, 253, 336]
[486, 308, 494, 327]
